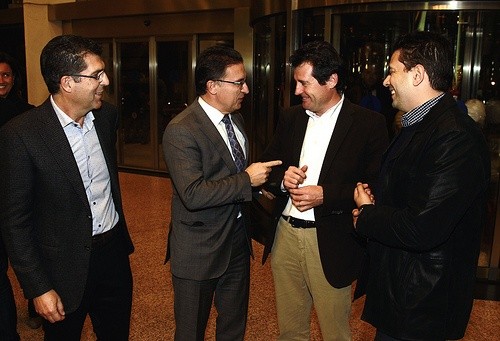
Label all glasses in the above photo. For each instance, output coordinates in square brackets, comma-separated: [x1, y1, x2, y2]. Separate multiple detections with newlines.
[213, 78, 249, 86]
[59, 68, 109, 84]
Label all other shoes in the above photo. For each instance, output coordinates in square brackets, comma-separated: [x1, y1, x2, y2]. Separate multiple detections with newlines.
[28, 315, 41, 328]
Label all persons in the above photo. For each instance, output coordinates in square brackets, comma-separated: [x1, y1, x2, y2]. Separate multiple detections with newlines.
[121, 49, 174, 144]
[164, 45, 283, 341]
[352, 31, 493, 341]
[0, 35, 134, 341]
[0, 59, 44, 341]
[339, 42, 486, 126]
[255, 39, 391, 341]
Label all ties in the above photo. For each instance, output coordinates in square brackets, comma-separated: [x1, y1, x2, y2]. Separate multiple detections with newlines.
[222, 112, 246, 176]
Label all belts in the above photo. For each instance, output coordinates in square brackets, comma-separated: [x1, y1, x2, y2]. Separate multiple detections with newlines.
[281, 213, 318, 229]
[91, 221, 124, 247]
